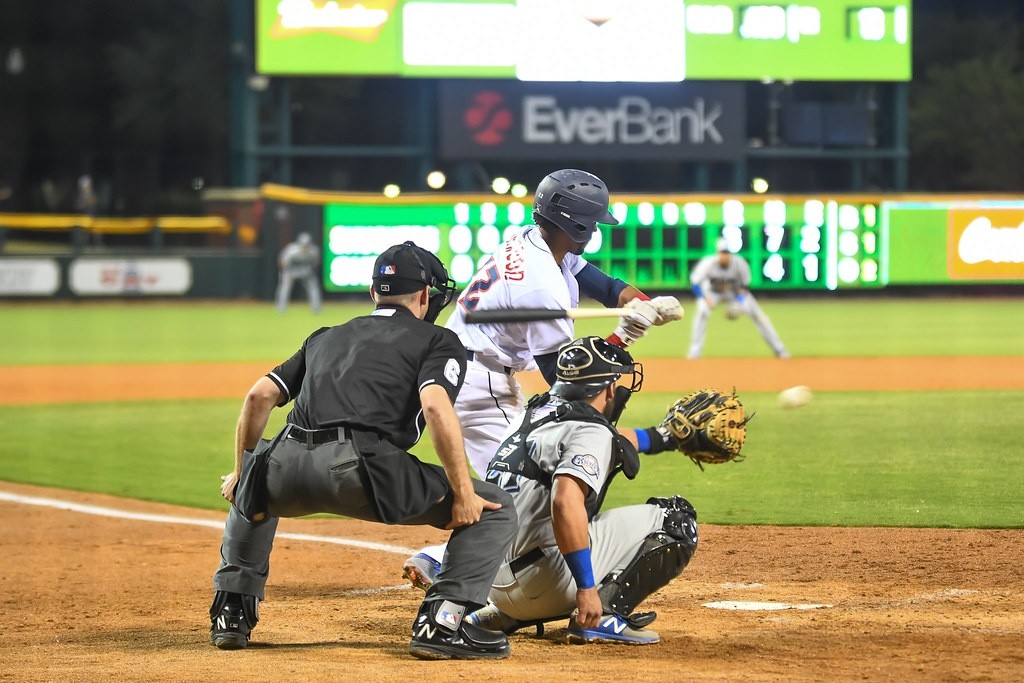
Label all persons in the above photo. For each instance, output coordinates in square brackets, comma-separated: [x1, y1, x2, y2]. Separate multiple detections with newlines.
[464, 335, 746, 644]
[276, 232, 320, 309]
[687, 239, 787, 359]
[210, 239, 519, 660]
[401, 169, 683, 610]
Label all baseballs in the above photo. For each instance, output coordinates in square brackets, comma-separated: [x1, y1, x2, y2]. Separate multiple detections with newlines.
[781, 386, 810, 410]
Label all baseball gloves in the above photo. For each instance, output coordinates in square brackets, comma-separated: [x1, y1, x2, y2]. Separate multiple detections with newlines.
[664, 389, 748, 464]
[726, 302, 744, 320]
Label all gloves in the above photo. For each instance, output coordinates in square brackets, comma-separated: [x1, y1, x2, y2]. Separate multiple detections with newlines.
[617, 297, 658, 340]
[650, 296, 683, 326]
[648, 421, 680, 456]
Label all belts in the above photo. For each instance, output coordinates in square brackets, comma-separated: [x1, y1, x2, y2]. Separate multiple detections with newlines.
[509, 547, 544, 574]
[283, 424, 351, 445]
[467, 349, 511, 375]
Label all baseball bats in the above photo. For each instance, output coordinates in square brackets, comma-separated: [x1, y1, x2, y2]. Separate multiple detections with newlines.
[462, 308, 627, 324]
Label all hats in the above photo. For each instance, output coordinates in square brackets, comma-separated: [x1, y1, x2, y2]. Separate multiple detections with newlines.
[372, 241, 432, 296]
[718, 240, 732, 253]
[549, 336, 634, 400]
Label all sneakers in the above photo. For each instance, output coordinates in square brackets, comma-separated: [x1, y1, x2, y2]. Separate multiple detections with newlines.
[209, 603, 249, 649]
[407, 600, 510, 659]
[402, 552, 442, 594]
[566, 612, 660, 644]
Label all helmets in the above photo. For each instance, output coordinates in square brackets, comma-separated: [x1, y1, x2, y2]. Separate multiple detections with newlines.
[532, 169, 619, 243]
